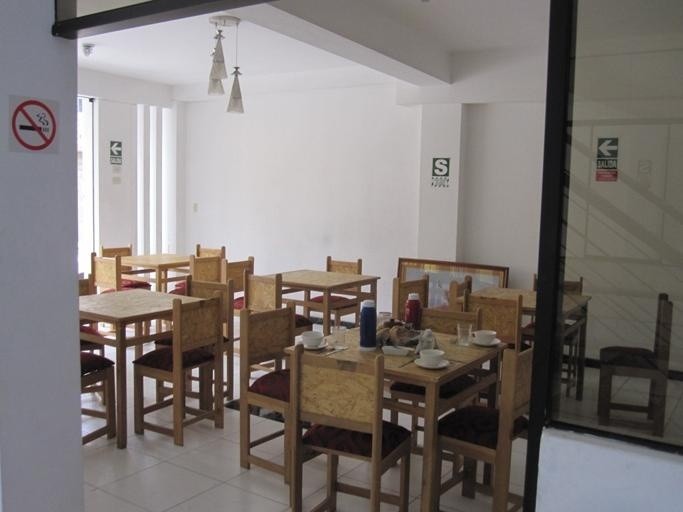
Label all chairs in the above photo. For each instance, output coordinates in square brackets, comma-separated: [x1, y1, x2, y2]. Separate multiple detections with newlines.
[598, 292, 673, 437]
[80, 241, 584, 512]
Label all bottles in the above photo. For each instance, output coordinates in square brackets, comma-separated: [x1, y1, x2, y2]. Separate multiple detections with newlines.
[378, 311, 393, 329]
[421, 329, 436, 349]
[404, 292, 422, 329]
[358, 299, 377, 351]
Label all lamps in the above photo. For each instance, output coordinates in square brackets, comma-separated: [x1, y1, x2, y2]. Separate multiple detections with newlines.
[208, 15, 244, 113]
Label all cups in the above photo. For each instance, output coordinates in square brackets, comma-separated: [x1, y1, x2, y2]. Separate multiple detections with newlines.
[455, 321, 471, 346]
[419, 349, 444, 366]
[302, 330, 324, 346]
[331, 326, 349, 352]
[472, 329, 498, 343]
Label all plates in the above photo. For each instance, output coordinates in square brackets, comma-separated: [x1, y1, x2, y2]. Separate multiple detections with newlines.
[466, 335, 502, 347]
[295, 340, 327, 351]
[412, 358, 451, 370]
